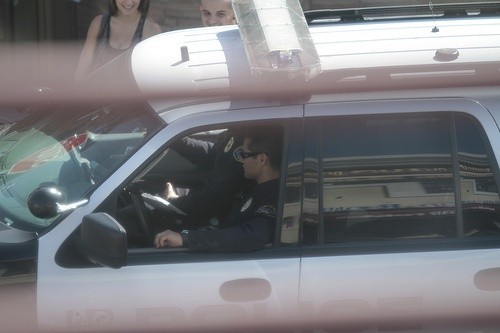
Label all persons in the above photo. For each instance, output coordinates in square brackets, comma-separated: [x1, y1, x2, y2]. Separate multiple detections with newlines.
[154, 129, 286, 250]
[76, 0, 161, 84]
[200, 0, 235, 28]
[159, 125, 277, 224]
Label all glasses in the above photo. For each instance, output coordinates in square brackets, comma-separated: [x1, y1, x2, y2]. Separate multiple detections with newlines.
[240, 151, 267, 159]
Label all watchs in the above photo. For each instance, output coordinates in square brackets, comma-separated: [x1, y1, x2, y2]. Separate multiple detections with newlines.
[181, 229, 190, 249]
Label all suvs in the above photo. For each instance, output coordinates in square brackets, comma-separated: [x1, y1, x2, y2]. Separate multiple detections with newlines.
[0, 0, 500, 333]
